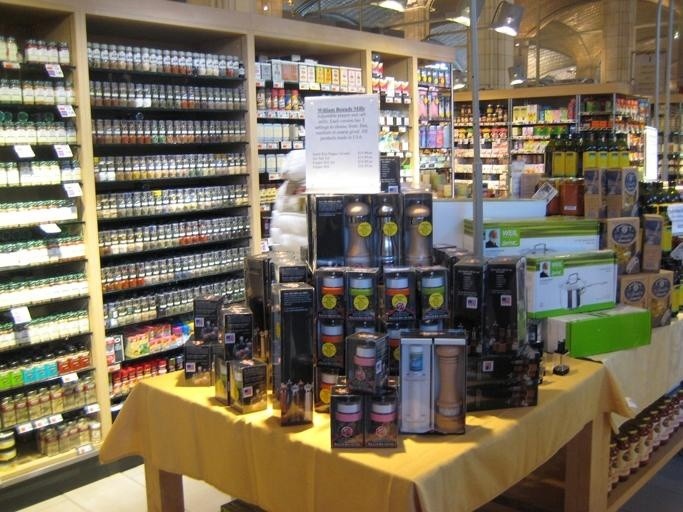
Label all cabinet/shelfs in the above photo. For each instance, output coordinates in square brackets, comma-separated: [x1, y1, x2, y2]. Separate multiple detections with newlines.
[0, 0, 456, 512]
[453, 83, 649, 191]
[642, 94, 683, 185]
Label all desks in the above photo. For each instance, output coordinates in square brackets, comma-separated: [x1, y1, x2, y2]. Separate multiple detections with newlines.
[583, 310, 683, 512]
[98, 351, 635, 512]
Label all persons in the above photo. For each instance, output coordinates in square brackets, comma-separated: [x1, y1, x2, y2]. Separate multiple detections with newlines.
[268, 149, 308, 268]
[540, 263, 550, 277]
[485, 230, 499, 248]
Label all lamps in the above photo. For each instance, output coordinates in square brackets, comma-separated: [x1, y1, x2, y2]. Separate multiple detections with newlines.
[457, 1, 485, 27]
[453, 70, 465, 90]
[508, 64, 526, 85]
[488, 1, 524, 36]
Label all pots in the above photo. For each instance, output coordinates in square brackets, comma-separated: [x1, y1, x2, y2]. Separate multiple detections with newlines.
[558, 271, 609, 310]
[517, 242, 557, 254]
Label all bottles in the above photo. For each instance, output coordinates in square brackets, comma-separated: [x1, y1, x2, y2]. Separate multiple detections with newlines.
[566, 135, 578, 176]
[321, 277, 344, 312]
[582, 132, 596, 178]
[0, 111, 77, 145]
[544, 134, 558, 176]
[655, 405, 669, 446]
[319, 322, 344, 355]
[353, 345, 378, 384]
[608, 454, 612, 496]
[631, 147, 644, 165]
[662, 399, 674, 438]
[0, 75, 75, 105]
[320, 371, 338, 404]
[422, 277, 445, 312]
[336, 401, 362, 439]
[552, 134, 566, 177]
[632, 423, 649, 466]
[616, 98, 639, 119]
[385, 328, 411, 363]
[611, 442, 619, 489]
[98, 216, 250, 257]
[94, 155, 247, 182]
[616, 133, 630, 167]
[639, 182, 683, 317]
[668, 394, 679, 433]
[0, 311, 90, 351]
[92, 119, 246, 144]
[0, 161, 81, 187]
[104, 278, 251, 329]
[101, 245, 252, 295]
[646, 410, 660, 451]
[0, 273, 88, 310]
[615, 435, 630, 482]
[639, 416, 653, 457]
[623, 428, 639, 473]
[348, 278, 374, 310]
[676, 388, 683, 426]
[0, 374, 97, 433]
[621, 124, 644, 143]
[90, 80, 247, 109]
[0, 37, 71, 66]
[95, 184, 249, 220]
[35, 416, 103, 457]
[0, 344, 91, 391]
[386, 277, 410, 312]
[0, 200, 77, 229]
[596, 132, 609, 169]
[87, 36, 246, 78]
[367, 402, 395, 439]
[375, 203, 397, 264]
[608, 133, 620, 168]
[107, 355, 184, 394]
[0, 236, 85, 269]
